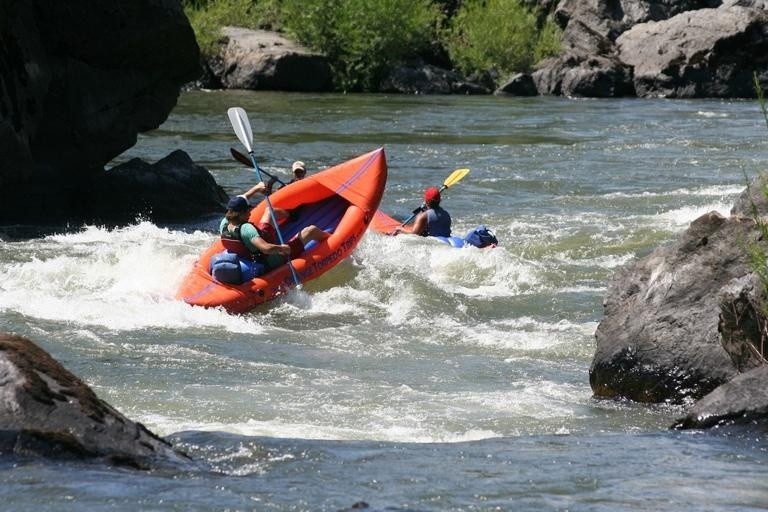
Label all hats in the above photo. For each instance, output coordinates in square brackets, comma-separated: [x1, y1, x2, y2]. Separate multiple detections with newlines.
[226, 194, 252, 212]
[424, 187, 442, 206]
[292, 160, 307, 173]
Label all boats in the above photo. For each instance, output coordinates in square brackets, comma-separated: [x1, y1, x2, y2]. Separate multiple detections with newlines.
[368, 207, 411, 239]
[172, 145, 390, 316]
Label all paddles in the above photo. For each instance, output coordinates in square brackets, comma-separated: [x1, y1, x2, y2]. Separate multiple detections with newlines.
[227, 106, 310, 306]
[391, 168, 470, 235]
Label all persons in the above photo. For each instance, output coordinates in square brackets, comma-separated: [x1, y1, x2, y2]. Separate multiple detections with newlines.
[219, 181, 332, 272]
[394, 186, 452, 237]
[276, 161, 308, 192]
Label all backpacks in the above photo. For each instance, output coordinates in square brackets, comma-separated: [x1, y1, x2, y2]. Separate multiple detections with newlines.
[466, 225, 498, 248]
